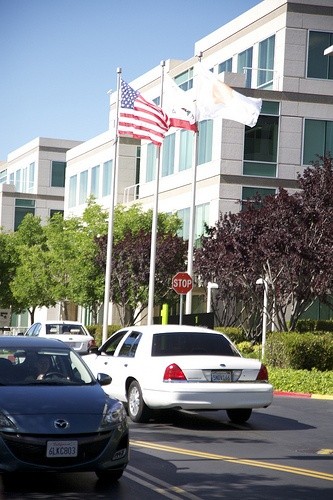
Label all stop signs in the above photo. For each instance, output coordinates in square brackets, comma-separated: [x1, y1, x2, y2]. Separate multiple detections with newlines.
[171, 271, 194, 296]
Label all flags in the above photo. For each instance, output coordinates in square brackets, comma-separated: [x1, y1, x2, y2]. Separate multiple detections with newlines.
[195, 60, 262, 128]
[146, 122, 148, 124]
[116, 79, 170, 145]
[162, 71, 199, 132]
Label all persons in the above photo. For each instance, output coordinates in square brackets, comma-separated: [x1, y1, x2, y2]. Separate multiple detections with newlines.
[24, 355, 70, 381]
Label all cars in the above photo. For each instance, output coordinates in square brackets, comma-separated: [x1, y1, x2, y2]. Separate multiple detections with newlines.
[0, 335, 130, 483]
[17, 320, 96, 355]
[77, 324, 274, 424]
